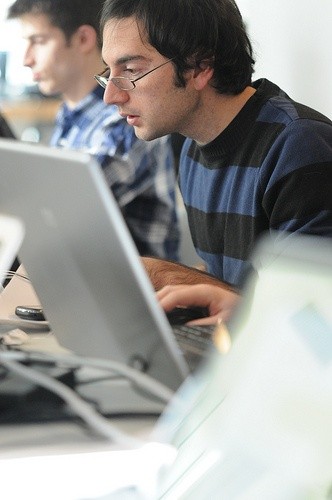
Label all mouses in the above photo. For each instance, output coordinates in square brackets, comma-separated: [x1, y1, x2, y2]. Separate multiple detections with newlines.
[165, 305, 210, 326]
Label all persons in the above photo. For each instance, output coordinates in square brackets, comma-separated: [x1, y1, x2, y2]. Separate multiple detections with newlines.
[0, 0, 181, 265]
[95, 0, 331, 289]
[155, 285, 242, 327]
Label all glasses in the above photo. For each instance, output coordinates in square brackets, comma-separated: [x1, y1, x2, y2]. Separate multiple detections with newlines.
[94, 55, 180, 91]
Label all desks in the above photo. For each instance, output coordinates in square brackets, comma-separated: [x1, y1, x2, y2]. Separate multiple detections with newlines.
[0, 240, 329, 499]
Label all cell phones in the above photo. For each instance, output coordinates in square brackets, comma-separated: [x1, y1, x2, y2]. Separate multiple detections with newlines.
[15, 305, 45, 321]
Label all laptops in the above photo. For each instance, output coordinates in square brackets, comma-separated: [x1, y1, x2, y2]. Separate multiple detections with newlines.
[0, 138, 217, 403]
